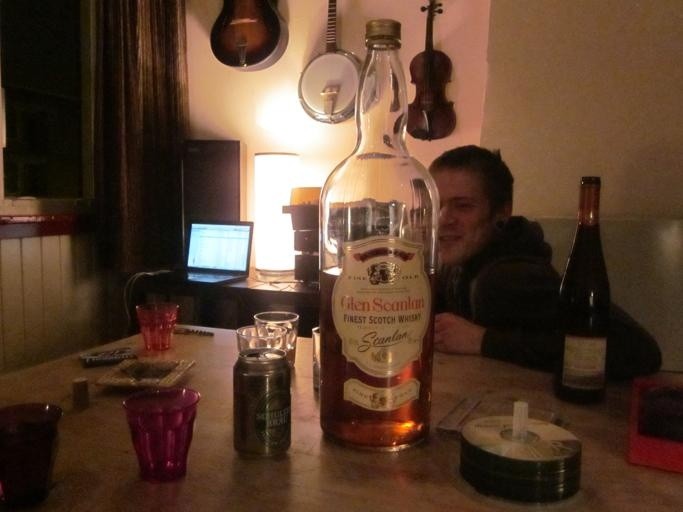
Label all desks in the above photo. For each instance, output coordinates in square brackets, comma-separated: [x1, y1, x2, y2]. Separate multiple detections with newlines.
[0, 323, 683, 512]
[130, 267, 318, 336]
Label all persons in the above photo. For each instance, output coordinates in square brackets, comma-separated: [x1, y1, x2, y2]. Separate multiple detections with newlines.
[425, 144, 663, 380]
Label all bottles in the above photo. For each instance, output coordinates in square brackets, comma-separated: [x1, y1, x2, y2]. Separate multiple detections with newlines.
[319, 19, 440, 456]
[554, 176, 611, 405]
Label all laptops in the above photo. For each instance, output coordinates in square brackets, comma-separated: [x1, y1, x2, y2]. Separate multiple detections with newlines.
[159, 220, 254, 286]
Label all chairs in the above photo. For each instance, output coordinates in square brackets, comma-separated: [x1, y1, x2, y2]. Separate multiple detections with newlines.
[609, 303, 663, 383]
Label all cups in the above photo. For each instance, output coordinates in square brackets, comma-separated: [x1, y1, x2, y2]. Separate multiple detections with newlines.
[0, 401, 63, 507]
[134, 301, 179, 350]
[237, 311, 299, 370]
[122, 388, 200, 480]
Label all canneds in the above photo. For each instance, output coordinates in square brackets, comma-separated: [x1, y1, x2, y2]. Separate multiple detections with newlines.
[233, 348, 292, 459]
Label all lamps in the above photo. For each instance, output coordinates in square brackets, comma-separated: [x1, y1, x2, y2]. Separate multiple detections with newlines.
[252, 150, 300, 276]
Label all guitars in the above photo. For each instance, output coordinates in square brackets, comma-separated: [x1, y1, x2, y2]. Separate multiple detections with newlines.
[210, 1, 288, 71]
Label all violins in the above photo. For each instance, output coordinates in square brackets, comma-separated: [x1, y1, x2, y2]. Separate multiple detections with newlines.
[406, 1, 455, 139]
